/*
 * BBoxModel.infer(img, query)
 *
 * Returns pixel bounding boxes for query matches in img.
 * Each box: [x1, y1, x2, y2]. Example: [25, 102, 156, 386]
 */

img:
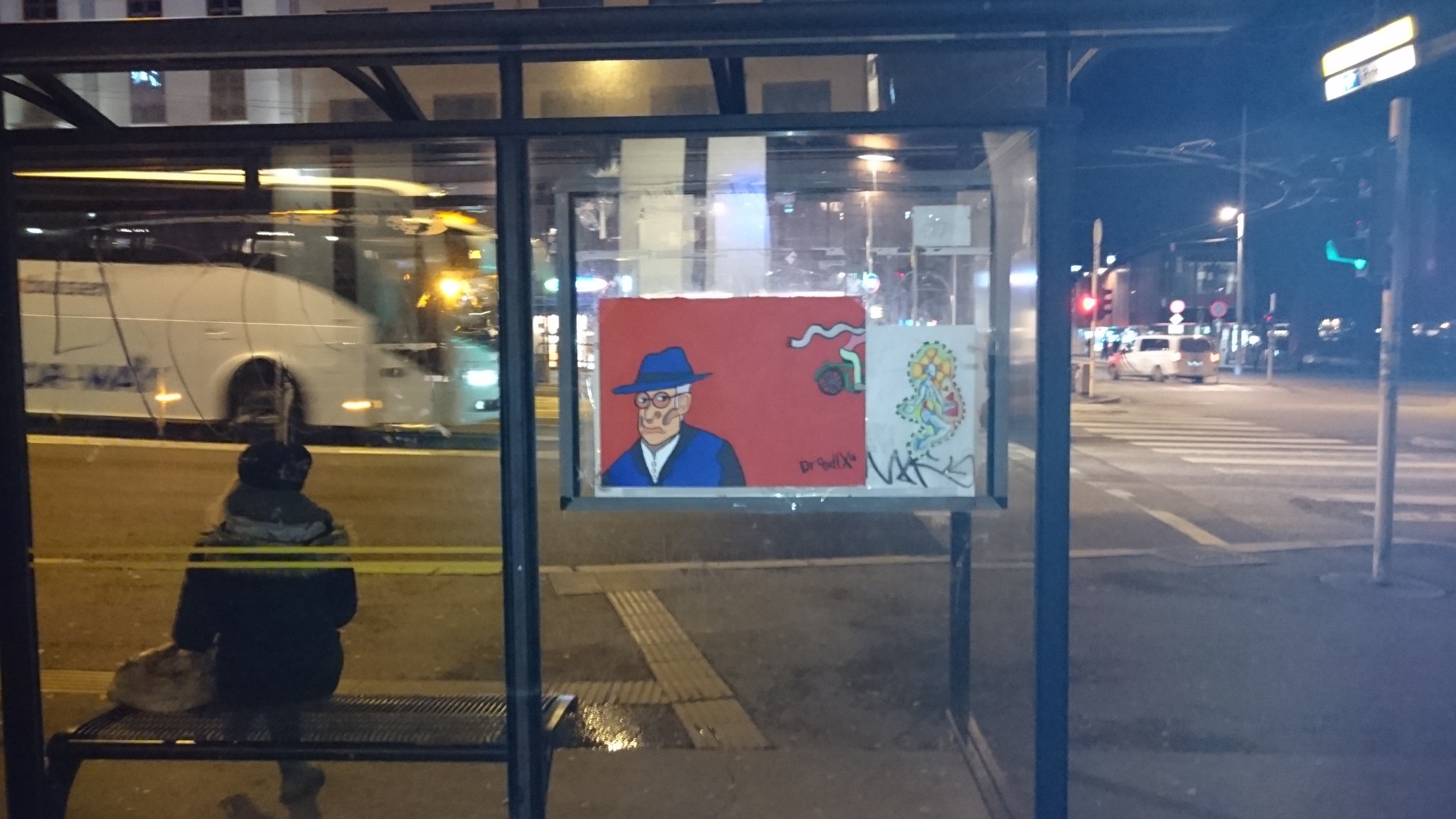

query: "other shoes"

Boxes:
[280, 765, 324, 800]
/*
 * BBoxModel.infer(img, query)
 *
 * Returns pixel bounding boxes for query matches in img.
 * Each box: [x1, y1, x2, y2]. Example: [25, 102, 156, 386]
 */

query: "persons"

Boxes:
[176, 448, 357, 809]
[1099, 340, 1109, 361]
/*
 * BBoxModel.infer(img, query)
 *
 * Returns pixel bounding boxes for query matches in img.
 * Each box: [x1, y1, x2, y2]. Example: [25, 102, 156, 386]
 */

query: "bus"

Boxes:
[1, 165, 562, 449]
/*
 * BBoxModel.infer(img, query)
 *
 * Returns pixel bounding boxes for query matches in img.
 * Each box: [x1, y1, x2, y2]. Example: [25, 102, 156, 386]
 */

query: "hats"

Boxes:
[237, 440, 312, 490]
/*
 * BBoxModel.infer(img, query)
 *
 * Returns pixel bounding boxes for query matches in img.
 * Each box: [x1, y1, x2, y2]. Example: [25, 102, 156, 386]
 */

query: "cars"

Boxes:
[1070, 326, 1161, 386]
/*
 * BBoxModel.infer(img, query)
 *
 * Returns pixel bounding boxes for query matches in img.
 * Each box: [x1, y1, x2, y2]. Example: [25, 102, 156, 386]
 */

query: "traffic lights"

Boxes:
[1096, 286, 1113, 326]
[1316, 138, 1394, 288]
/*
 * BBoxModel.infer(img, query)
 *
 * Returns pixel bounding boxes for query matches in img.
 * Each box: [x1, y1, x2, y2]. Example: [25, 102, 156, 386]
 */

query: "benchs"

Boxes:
[47, 692, 580, 819]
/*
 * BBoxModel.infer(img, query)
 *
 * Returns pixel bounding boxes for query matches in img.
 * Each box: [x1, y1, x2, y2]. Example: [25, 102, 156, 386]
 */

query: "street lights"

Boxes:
[1317, 15, 1421, 584]
[1216, 206, 1247, 374]
[1069, 216, 1115, 398]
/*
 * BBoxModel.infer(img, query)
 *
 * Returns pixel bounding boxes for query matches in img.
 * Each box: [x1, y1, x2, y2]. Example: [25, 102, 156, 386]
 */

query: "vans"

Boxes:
[1105, 333, 1219, 384]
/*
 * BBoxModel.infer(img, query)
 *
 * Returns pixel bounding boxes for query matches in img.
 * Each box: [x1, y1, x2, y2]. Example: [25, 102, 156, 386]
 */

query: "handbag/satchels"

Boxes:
[107, 641, 216, 712]
